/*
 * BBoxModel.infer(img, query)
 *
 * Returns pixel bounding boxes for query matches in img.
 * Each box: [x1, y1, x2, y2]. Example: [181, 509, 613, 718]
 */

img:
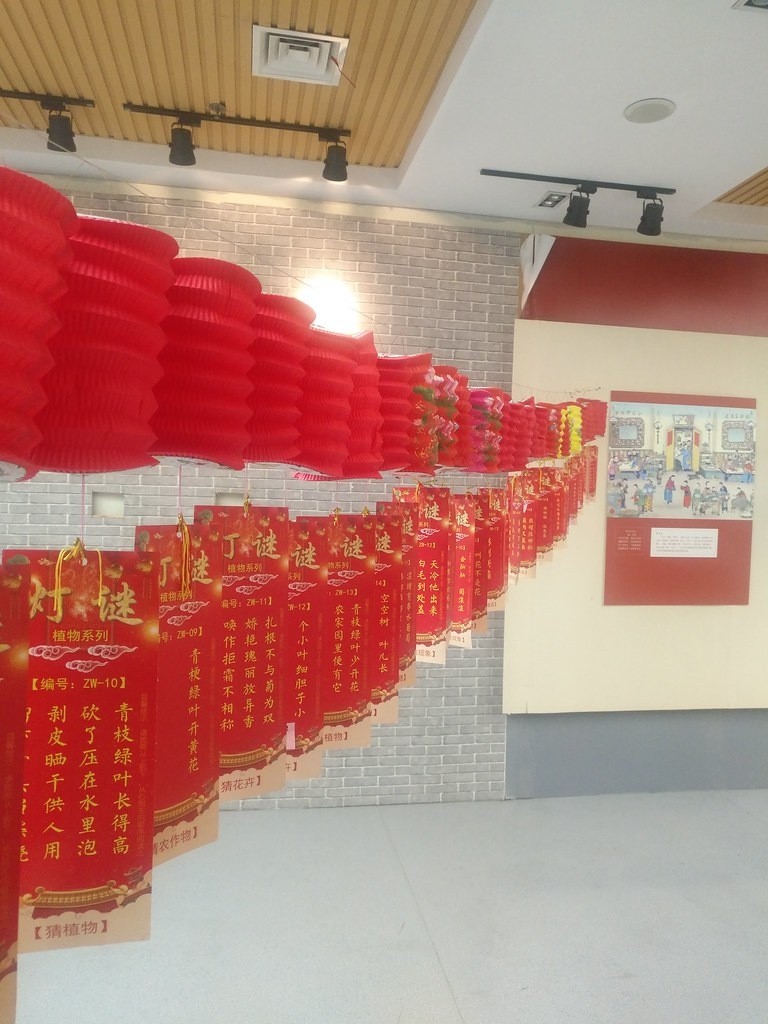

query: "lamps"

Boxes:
[168, 115, 200, 166]
[40, 100, 76, 152]
[317, 132, 348, 181]
[562, 183, 596, 229]
[634, 188, 664, 236]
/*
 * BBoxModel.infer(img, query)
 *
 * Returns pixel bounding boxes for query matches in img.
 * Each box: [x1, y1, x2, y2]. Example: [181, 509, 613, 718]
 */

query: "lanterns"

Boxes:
[0, 165, 608, 481]
